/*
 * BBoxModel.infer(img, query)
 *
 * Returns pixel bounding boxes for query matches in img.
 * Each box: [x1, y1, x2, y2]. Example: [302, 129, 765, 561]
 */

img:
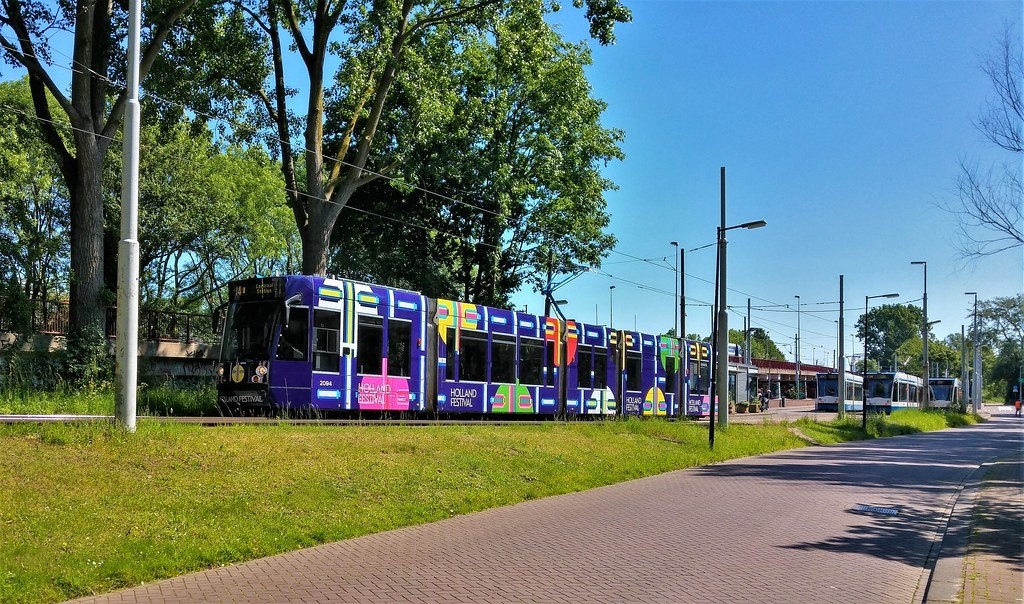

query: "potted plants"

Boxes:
[736, 400, 749, 413]
[748, 399, 762, 413]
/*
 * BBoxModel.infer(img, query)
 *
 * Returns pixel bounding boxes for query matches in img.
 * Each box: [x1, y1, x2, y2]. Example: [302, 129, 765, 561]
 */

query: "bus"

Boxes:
[862, 369, 923, 414]
[211, 266, 719, 419]
[814, 367, 863, 412]
[929, 377, 963, 411]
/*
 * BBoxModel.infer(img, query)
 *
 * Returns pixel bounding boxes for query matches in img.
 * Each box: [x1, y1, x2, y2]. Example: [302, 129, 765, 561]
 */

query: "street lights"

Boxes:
[610, 286, 616, 328]
[821, 345, 825, 365]
[964, 292, 978, 414]
[709, 219, 767, 449]
[670, 241, 679, 337]
[813, 348, 816, 365]
[795, 295, 801, 400]
[910, 261, 930, 409]
[862, 293, 900, 429]
[835, 321, 842, 375]
[851, 333, 854, 374]
[1019, 366, 1024, 403]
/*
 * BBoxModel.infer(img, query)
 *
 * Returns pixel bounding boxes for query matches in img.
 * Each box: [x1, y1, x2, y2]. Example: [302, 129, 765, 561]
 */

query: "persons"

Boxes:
[1014, 399, 1023, 416]
[758, 388, 772, 412]
